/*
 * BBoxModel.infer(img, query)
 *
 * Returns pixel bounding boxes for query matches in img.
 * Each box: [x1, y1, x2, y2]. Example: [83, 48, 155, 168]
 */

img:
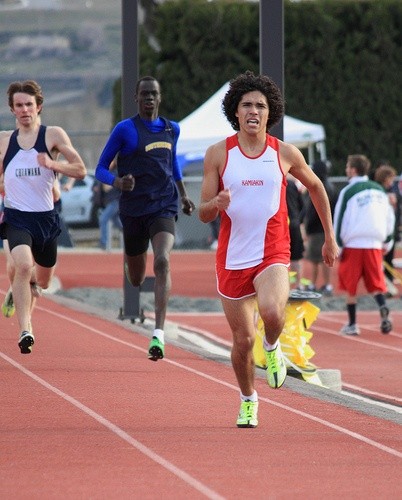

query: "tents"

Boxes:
[170, 79, 328, 249]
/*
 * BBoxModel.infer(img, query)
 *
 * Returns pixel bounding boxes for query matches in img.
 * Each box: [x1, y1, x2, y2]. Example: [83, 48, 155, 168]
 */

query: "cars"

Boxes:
[58, 169, 104, 228]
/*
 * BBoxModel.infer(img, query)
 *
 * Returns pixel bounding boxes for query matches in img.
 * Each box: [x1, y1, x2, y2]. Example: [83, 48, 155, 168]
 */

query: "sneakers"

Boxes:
[2, 287, 17, 317]
[236, 400, 259, 428]
[379, 304, 392, 334]
[338, 325, 360, 337]
[264, 334, 287, 389]
[146, 336, 165, 362]
[18, 331, 35, 354]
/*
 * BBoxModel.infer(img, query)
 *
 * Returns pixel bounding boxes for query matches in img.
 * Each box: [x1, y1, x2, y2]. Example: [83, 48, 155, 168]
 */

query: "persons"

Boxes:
[0, 81, 86, 354]
[372, 164, 397, 297]
[0, 108, 75, 318]
[267, 161, 309, 295]
[301, 160, 336, 292]
[92, 157, 124, 250]
[94, 74, 196, 362]
[196, 72, 340, 427]
[331, 154, 394, 335]
